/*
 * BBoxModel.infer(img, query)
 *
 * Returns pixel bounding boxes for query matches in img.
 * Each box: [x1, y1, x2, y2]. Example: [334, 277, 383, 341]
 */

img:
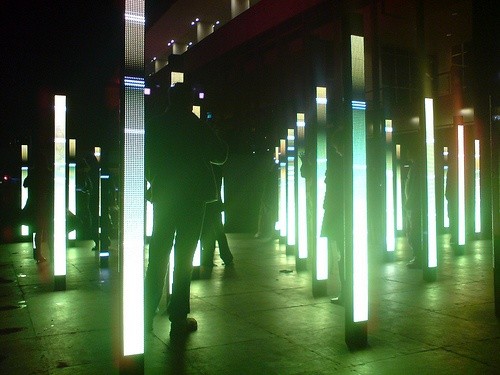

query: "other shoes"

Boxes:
[330, 297, 341, 305]
[170, 317, 198, 338]
[407, 256, 418, 268]
[92, 245, 99, 251]
[224, 259, 233, 265]
[203, 265, 213, 278]
[253, 232, 267, 239]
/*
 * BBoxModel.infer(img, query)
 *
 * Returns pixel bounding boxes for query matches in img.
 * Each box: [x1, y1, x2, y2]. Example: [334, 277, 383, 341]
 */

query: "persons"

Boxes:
[14, 147, 115, 263]
[202, 104, 466, 304]
[143, 82, 230, 338]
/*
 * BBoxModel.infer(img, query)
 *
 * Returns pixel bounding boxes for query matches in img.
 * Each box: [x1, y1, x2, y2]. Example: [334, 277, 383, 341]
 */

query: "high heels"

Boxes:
[34, 256, 47, 264]
